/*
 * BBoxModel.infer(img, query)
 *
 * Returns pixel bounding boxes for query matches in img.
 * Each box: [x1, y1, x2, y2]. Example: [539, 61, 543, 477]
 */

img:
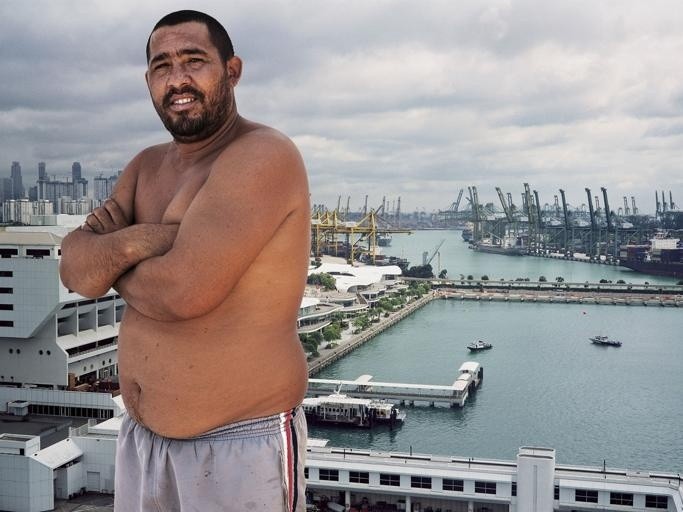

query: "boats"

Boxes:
[467, 340, 492, 352]
[302, 393, 407, 428]
[619, 231, 683, 277]
[366, 232, 392, 246]
[473, 230, 528, 256]
[588, 335, 622, 347]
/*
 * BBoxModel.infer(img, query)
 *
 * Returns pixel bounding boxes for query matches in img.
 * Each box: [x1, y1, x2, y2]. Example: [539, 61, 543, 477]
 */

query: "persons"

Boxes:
[55, 8, 311, 512]
